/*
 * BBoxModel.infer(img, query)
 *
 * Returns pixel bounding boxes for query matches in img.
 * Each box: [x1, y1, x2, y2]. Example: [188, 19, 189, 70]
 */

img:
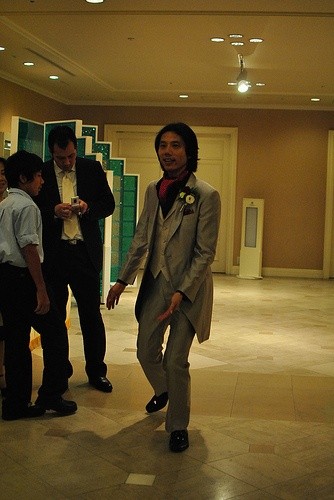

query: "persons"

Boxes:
[0, 150, 78, 421]
[106, 122, 222, 453]
[31, 126, 116, 396]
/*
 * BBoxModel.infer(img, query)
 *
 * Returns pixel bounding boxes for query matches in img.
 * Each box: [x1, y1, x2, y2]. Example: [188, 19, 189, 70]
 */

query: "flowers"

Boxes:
[178, 191, 195, 206]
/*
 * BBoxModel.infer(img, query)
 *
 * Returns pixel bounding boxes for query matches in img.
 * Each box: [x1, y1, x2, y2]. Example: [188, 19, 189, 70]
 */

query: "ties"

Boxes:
[61, 170, 80, 240]
[157, 175, 178, 201]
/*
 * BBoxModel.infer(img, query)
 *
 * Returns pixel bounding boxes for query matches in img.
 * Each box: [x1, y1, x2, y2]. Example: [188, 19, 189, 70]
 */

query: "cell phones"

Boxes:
[70, 196, 79, 206]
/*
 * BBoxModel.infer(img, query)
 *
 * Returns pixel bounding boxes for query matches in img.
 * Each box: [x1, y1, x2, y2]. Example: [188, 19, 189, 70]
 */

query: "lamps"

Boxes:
[236, 54, 249, 93]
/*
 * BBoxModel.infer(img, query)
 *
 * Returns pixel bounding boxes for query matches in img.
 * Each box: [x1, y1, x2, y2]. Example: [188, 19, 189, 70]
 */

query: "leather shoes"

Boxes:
[88, 375, 113, 393]
[35, 394, 77, 415]
[145, 392, 168, 413]
[169, 430, 189, 452]
[60, 378, 68, 394]
[2, 400, 42, 420]
